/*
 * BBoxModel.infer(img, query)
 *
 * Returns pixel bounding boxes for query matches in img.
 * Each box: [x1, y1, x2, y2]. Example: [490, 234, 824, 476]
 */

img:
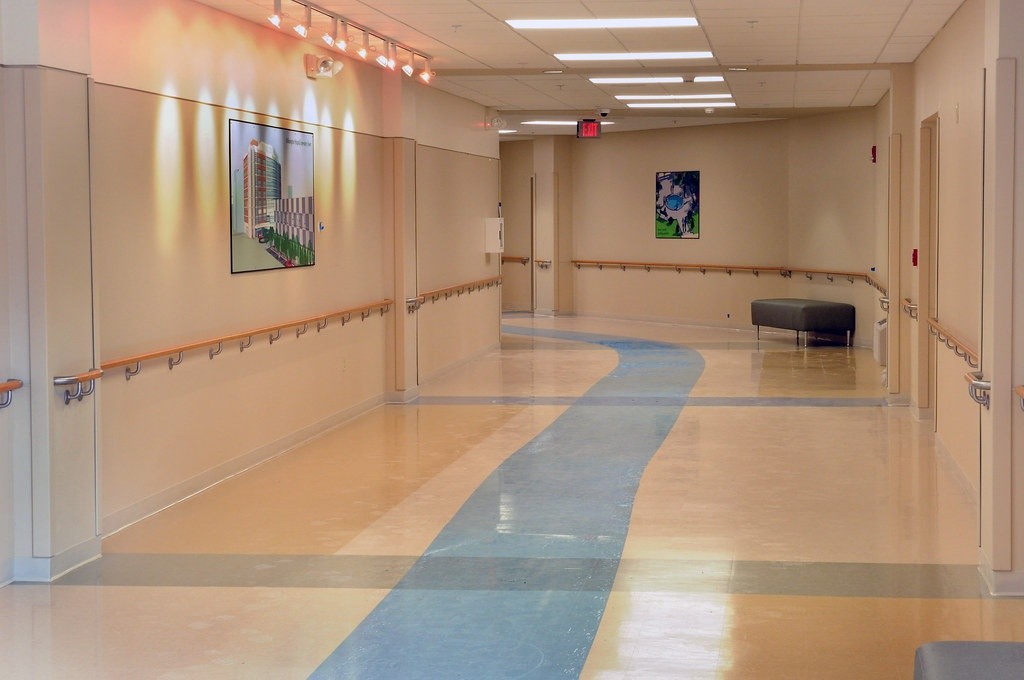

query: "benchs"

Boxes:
[751, 298, 856, 347]
[912, 641, 1024, 680]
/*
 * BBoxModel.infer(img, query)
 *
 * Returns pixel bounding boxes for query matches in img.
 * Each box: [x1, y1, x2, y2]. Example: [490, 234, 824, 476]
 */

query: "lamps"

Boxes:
[376, 40, 389, 66]
[388, 44, 398, 70]
[402, 52, 415, 77]
[268, 0, 285, 28]
[304, 54, 344, 79]
[322, 18, 338, 48]
[293, 6, 312, 38]
[356, 31, 370, 59]
[336, 21, 349, 52]
[419, 59, 436, 84]
